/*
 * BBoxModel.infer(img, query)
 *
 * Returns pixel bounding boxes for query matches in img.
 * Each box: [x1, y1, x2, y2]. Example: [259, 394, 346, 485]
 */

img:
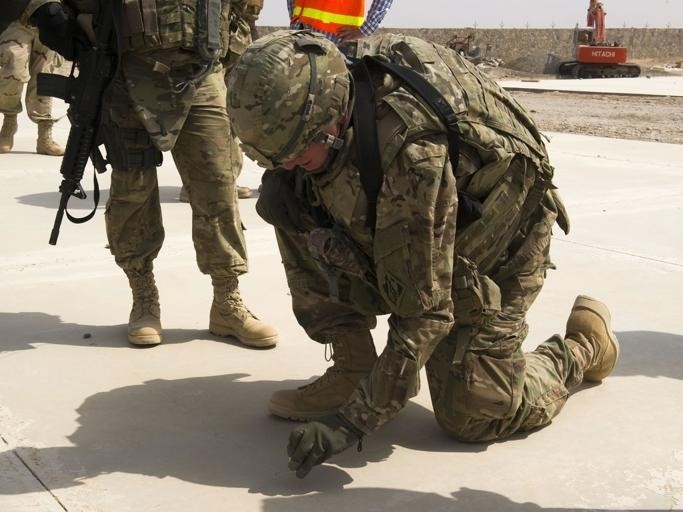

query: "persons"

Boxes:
[1, 1, 66, 154]
[180, 0, 264, 203]
[9, 0, 279, 348]
[225, 26, 620, 477]
[259, 0, 392, 192]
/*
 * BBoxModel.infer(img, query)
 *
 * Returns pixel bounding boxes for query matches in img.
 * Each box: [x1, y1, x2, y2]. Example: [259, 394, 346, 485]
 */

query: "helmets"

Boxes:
[225, 28, 351, 170]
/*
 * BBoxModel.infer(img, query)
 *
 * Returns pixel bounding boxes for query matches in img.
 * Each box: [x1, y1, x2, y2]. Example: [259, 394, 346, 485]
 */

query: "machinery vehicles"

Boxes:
[554, 1, 641, 78]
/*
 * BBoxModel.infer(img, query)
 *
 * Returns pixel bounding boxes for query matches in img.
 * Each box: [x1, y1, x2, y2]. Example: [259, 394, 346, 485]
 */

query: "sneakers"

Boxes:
[180, 179, 263, 203]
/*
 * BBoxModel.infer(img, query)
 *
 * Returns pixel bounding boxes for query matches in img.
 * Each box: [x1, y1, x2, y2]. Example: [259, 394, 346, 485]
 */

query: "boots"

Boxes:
[0, 113, 18, 153]
[36, 121, 65, 155]
[126, 271, 164, 347]
[208, 276, 280, 348]
[563, 294, 620, 383]
[268, 327, 379, 423]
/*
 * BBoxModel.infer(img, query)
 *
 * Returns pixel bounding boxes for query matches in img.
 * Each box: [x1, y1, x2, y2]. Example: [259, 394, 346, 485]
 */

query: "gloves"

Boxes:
[37, 2, 76, 62]
[286, 412, 364, 479]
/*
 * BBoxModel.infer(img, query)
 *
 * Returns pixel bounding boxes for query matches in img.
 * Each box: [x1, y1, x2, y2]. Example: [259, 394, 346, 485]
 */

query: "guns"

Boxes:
[36, 0, 122, 245]
